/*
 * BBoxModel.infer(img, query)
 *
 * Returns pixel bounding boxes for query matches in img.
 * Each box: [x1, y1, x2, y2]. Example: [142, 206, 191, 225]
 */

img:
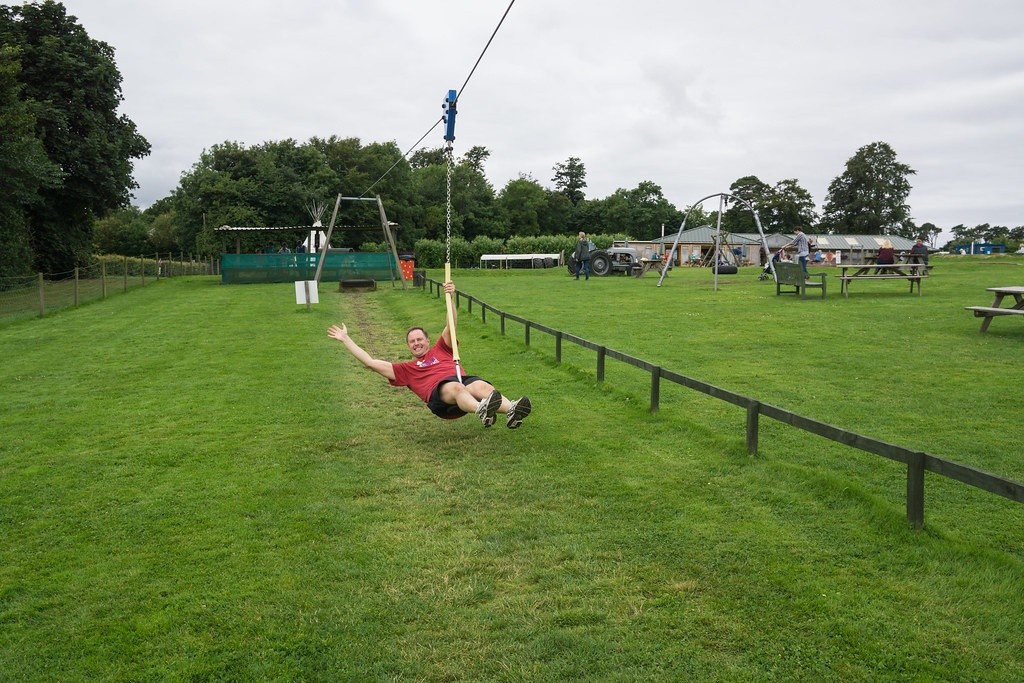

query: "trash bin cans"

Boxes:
[399, 254, 415, 279]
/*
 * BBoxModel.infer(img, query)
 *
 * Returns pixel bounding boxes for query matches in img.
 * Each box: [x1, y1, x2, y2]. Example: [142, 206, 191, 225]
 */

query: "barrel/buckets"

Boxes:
[399, 255, 415, 280]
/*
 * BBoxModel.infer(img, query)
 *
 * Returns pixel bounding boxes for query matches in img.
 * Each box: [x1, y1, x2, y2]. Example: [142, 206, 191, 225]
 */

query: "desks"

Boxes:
[854, 256, 907, 276]
[637, 260, 668, 279]
[837, 264, 934, 296]
[893, 254, 925, 268]
[980, 287, 1024, 332]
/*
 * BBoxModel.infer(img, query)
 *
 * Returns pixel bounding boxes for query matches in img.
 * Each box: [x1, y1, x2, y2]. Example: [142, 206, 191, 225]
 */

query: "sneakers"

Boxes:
[477, 390, 502, 428]
[506, 396, 532, 429]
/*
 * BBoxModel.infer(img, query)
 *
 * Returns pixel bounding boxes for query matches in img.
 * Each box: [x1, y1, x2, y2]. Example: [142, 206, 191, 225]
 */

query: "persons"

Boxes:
[909, 238, 929, 276]
[327, 281, 532, 430]
[254, 239, 308, 278]
[649, 251, 697, 267]
[1020, 243, 1024, 254]
[873, 240, 894, 275]
[574, 232, 591, 281]
[778, 225, 834, 279]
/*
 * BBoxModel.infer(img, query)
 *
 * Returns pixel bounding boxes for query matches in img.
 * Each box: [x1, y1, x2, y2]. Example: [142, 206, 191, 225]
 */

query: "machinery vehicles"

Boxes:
[568, 237, 643, 279]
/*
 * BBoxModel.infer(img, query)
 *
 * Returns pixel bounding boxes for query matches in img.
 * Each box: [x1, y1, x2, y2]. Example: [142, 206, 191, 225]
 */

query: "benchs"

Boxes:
[632, 267, 642, 269]
[663, 266, 672, 269]
[774, 259, 827, 300]
[964, 306, 1024, 315]
[834, 274, 929, 279]
